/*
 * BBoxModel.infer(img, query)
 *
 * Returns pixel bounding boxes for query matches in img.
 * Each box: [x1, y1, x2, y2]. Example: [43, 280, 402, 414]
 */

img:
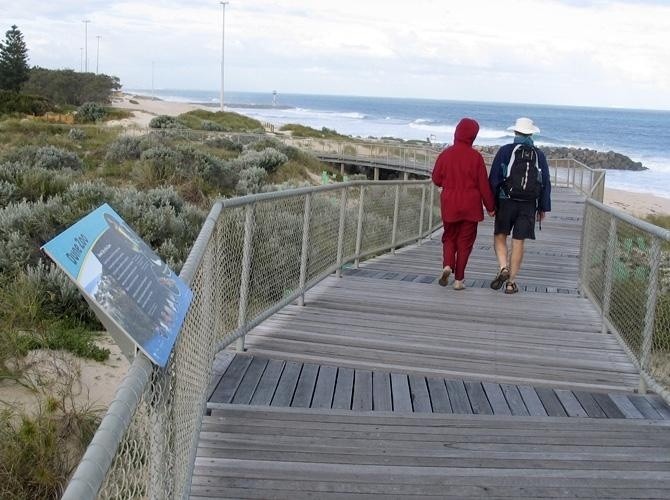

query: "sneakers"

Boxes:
[490, 266, 511, 289]
[507, 117, 541, 136]
[504, 281, 519, 293]
[453, 280, 467, 290]
[438, 266, 452, 287]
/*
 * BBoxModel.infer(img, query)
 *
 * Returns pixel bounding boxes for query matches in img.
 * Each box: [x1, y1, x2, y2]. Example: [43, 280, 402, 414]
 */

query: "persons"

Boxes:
[432, 118, 495, 290]
[489, 118, 551, 294]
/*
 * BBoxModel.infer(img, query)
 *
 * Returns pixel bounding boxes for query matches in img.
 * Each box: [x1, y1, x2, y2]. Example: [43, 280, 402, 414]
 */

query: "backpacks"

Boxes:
[498, 146, 544, 201]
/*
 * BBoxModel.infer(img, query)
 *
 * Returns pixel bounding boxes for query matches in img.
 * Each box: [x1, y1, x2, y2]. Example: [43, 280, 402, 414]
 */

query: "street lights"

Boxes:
[78, 46, 84, 71]
[81, 19, 91, 73]
[95, 35, 102, 74]
[216, 0, 230, 111]
